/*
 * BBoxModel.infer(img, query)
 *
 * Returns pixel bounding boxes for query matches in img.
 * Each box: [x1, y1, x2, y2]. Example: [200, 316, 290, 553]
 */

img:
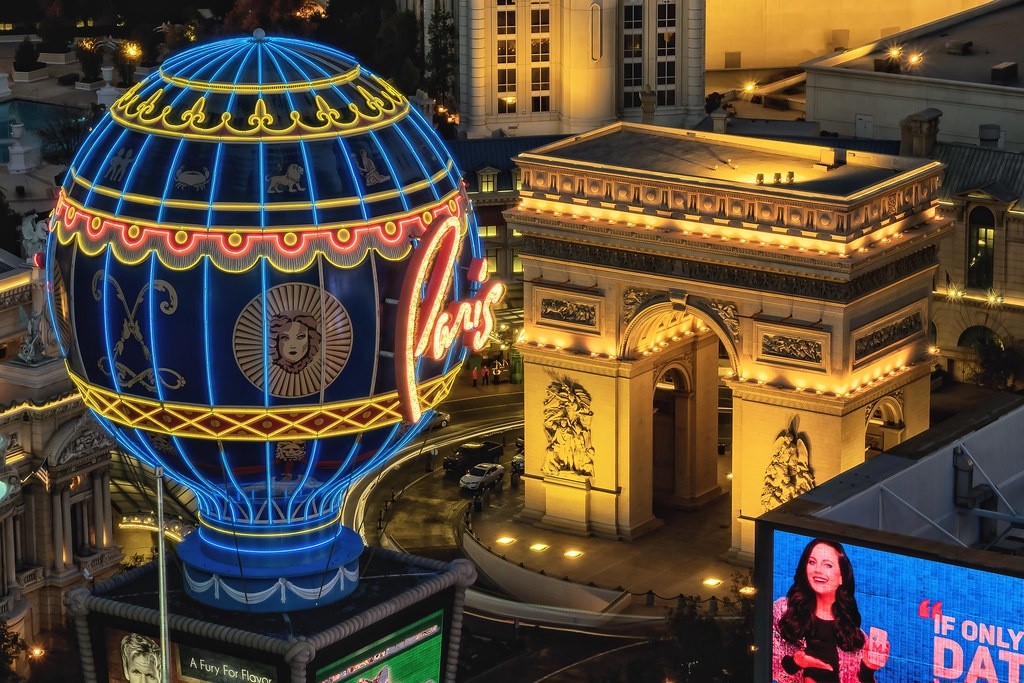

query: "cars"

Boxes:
[420, 410, 451, 433]
[460, 463, 504, 494]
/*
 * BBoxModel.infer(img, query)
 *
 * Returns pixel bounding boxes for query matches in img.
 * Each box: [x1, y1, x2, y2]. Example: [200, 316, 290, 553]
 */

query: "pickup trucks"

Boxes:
[443, 440, 504, 475]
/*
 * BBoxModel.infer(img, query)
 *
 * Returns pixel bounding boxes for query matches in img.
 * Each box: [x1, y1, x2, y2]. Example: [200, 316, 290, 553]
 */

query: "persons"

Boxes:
[772, 538, 890, 683]
[481, 365, 490, 386]
[120, 633, 162, 683]
[472, 366, 479, 387]
